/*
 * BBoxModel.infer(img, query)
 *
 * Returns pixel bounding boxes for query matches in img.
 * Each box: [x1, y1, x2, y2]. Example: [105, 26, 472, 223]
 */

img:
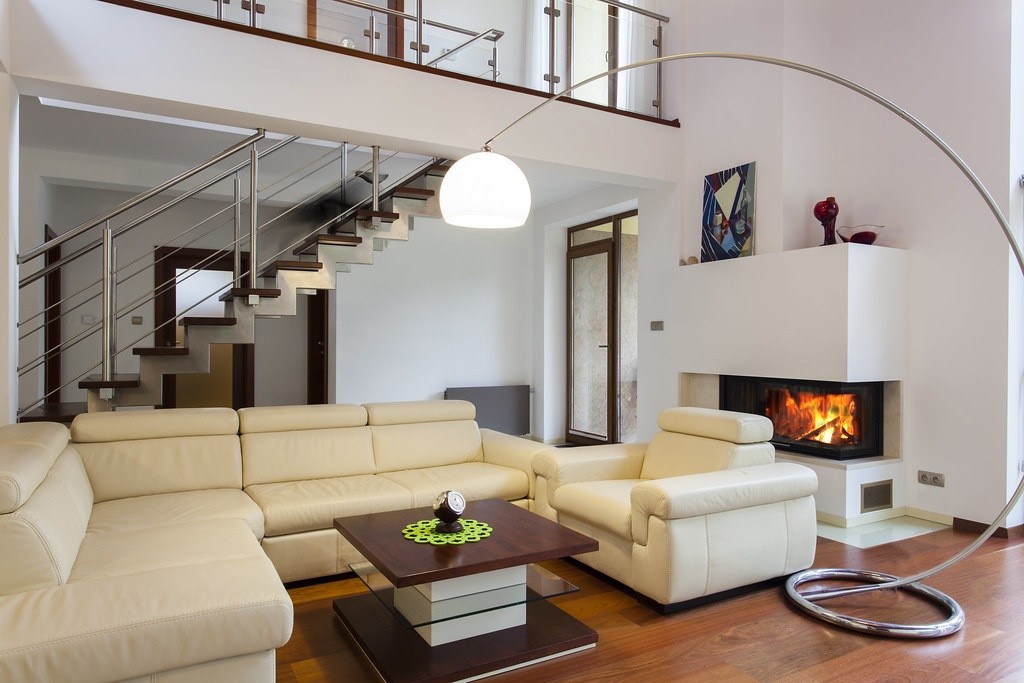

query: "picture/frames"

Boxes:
[700, 160, 757, 263]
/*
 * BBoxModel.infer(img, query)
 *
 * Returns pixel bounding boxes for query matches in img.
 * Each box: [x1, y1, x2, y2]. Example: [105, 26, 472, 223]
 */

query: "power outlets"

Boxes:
[917, 470, 945, 488]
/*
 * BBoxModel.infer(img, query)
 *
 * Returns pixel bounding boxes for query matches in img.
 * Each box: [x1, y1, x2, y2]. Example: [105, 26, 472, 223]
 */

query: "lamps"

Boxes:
[439, 52, 1024, 638]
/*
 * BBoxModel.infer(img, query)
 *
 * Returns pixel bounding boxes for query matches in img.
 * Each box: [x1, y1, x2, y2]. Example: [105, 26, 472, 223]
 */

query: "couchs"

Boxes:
[0, 394, 817, 683]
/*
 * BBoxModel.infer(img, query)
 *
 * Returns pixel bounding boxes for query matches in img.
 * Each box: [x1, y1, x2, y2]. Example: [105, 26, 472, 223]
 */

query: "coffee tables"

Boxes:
[332, 497, 599, 683]
